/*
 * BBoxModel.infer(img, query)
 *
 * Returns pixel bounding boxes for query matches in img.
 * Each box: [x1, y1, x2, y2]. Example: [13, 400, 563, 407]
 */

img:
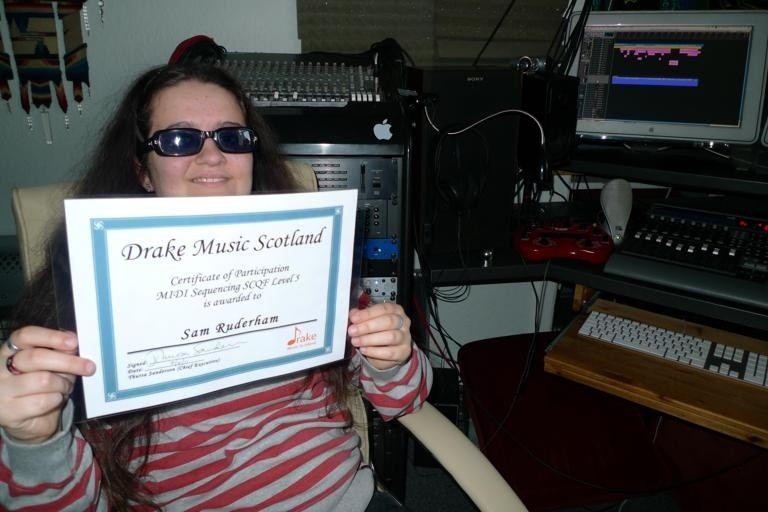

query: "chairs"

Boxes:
[6, 159, 537, 512]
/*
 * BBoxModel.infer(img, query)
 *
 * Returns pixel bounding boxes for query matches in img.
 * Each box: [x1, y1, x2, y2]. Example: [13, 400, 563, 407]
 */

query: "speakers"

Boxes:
[409, 67, 523, 269]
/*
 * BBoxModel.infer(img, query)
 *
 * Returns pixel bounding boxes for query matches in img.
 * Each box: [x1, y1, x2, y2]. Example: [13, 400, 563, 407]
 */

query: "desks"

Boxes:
[507, 199, 765, 451]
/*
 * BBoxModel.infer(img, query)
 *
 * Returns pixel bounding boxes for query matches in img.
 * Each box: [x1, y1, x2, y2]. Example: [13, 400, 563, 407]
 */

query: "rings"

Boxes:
[396, 313, 407, 332]
[5, 332, 22, 352]
[4, 352, 26, 373]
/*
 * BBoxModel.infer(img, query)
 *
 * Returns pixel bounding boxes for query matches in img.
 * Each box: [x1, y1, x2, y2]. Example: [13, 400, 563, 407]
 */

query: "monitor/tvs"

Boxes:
[569, 9, 768, 144]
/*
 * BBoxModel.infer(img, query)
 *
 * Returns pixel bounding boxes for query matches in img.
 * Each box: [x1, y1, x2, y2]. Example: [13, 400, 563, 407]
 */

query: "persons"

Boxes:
[2, 62, 442, 511]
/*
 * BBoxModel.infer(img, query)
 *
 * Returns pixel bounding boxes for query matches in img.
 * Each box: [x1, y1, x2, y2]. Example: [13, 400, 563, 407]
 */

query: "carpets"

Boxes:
[456, 331, 765, 510]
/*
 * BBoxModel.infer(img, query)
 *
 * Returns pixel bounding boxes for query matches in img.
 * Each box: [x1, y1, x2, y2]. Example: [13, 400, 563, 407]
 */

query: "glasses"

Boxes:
[144, 125, 261, 157]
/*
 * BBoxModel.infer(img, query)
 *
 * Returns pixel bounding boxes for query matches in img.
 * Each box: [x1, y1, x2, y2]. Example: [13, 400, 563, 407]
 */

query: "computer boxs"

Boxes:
[415, 368, 460, 466]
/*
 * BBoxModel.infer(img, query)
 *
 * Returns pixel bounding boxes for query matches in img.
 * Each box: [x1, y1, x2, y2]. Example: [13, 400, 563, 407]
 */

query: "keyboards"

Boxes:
[578, 311, 768, 389]
